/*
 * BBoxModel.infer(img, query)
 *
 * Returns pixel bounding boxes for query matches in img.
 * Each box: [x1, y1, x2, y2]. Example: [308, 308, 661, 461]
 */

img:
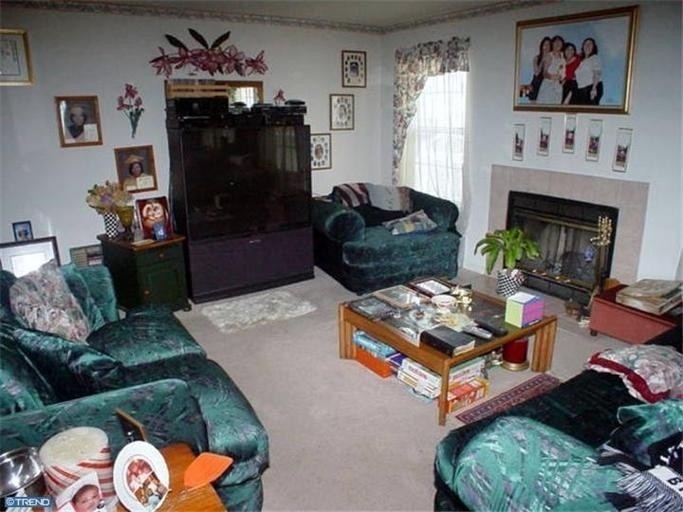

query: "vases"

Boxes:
[101, 209, 119, 239]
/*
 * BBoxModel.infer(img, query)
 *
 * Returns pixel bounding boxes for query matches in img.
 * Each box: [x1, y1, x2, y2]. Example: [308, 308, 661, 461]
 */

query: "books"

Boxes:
[418, 325, 476, 357]
[614, 278, 682, 317]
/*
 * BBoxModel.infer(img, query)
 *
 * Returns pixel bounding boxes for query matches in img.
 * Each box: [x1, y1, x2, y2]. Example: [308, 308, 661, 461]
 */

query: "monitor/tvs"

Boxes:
[165, 114, 312, 242]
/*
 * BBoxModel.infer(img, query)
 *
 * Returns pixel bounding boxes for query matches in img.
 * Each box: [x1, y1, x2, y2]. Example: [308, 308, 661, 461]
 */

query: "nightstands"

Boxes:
[97, 232, 191, 318]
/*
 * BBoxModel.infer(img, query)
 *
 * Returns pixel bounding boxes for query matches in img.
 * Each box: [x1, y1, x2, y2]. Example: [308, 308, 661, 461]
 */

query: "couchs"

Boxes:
[0, 263, 270, 511]
[433, 322, 683, 511]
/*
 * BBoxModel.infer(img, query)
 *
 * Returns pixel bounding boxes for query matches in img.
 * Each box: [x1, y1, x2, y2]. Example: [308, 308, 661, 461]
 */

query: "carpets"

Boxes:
[454, 372, 564, 426]
[202, 288, 319, 334]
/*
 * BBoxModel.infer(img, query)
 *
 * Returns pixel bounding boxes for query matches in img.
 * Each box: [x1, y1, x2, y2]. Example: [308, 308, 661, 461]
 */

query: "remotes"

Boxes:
[463, 325, 492, 339]
[477, 322, 508, 336]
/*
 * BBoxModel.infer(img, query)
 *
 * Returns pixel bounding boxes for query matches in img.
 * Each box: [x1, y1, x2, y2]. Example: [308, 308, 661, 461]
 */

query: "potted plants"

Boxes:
[473, 225, 542, 297]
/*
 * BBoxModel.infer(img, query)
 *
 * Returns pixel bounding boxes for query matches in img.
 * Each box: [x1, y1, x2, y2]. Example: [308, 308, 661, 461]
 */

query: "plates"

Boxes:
[431, 294, 456, 305]
[110, 439, 171, 512]
[408, 309, 432, 323]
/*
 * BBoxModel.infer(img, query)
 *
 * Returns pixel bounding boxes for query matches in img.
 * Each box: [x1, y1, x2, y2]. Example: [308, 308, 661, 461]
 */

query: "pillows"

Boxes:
[584, 343, 683, 403]
[13, 258, 126, 393]
[606, 400, 683, 466]
[382, 209, 438, 236]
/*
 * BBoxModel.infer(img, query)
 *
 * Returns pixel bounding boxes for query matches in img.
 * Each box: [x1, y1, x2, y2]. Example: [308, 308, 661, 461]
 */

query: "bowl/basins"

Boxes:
[0, 446, 47, 512]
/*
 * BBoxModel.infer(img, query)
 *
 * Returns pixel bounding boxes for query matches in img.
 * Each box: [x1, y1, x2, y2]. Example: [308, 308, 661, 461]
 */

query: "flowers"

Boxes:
[148, 27, 269, 78]
[116, 83, 145, 138]
[84, 179, 132, 215]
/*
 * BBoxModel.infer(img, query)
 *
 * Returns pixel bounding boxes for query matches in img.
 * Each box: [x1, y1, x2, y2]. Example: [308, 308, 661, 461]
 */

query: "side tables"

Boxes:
[30, 442, 227, 512]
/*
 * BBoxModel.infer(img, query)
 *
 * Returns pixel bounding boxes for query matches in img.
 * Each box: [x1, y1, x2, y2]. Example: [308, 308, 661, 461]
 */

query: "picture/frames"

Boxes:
[1, 28, 36, 87]
[512, 4, 639, 115]
[114, 144, 158, 193]
[347, 296, 397, 322]
[407, 274, 453, 298]
[373, 283, 430, 312]
[328, 93, 355, 131]
[12, 220, 33, 241]
[311, 132, 333, 170]
[115, 407, 147, 440]
[511, 114, 633, 173]
[1, 235, 61, 280]
[341, 49, 367, 88]
[53, 93, 103, 148]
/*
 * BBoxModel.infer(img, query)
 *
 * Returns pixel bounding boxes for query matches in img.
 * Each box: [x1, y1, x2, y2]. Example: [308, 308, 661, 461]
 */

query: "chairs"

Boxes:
[312, 181, 462, 296]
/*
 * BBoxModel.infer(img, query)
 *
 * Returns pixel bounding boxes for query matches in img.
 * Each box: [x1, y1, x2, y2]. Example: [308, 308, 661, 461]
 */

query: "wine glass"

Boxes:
[114, 205, 135, 241]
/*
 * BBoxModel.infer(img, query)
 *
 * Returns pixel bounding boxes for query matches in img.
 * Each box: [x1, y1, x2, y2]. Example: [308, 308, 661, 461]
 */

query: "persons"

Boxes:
[559, 42, 583, 106]
[573, 38, 603, 106]
[64, 105, 87, 138]
[70, 485, 100, 510]
[120, 154, 146, 189]
[535, 33, 567, 105]
[352, 329, 485, 399]
[125, 462, 166, 510]
[518, 34, 564, 104]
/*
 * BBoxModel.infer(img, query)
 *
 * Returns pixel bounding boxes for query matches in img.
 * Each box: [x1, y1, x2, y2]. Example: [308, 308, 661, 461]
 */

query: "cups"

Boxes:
[457, 288, 473, 316]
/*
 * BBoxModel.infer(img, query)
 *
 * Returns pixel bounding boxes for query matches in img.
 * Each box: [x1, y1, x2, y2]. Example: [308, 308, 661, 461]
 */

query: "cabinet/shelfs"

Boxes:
[165, 114, 315, 304]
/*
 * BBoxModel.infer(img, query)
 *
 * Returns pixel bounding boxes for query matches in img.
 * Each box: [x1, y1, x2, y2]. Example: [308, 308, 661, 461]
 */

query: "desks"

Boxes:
[588, 284, 683, 344]
